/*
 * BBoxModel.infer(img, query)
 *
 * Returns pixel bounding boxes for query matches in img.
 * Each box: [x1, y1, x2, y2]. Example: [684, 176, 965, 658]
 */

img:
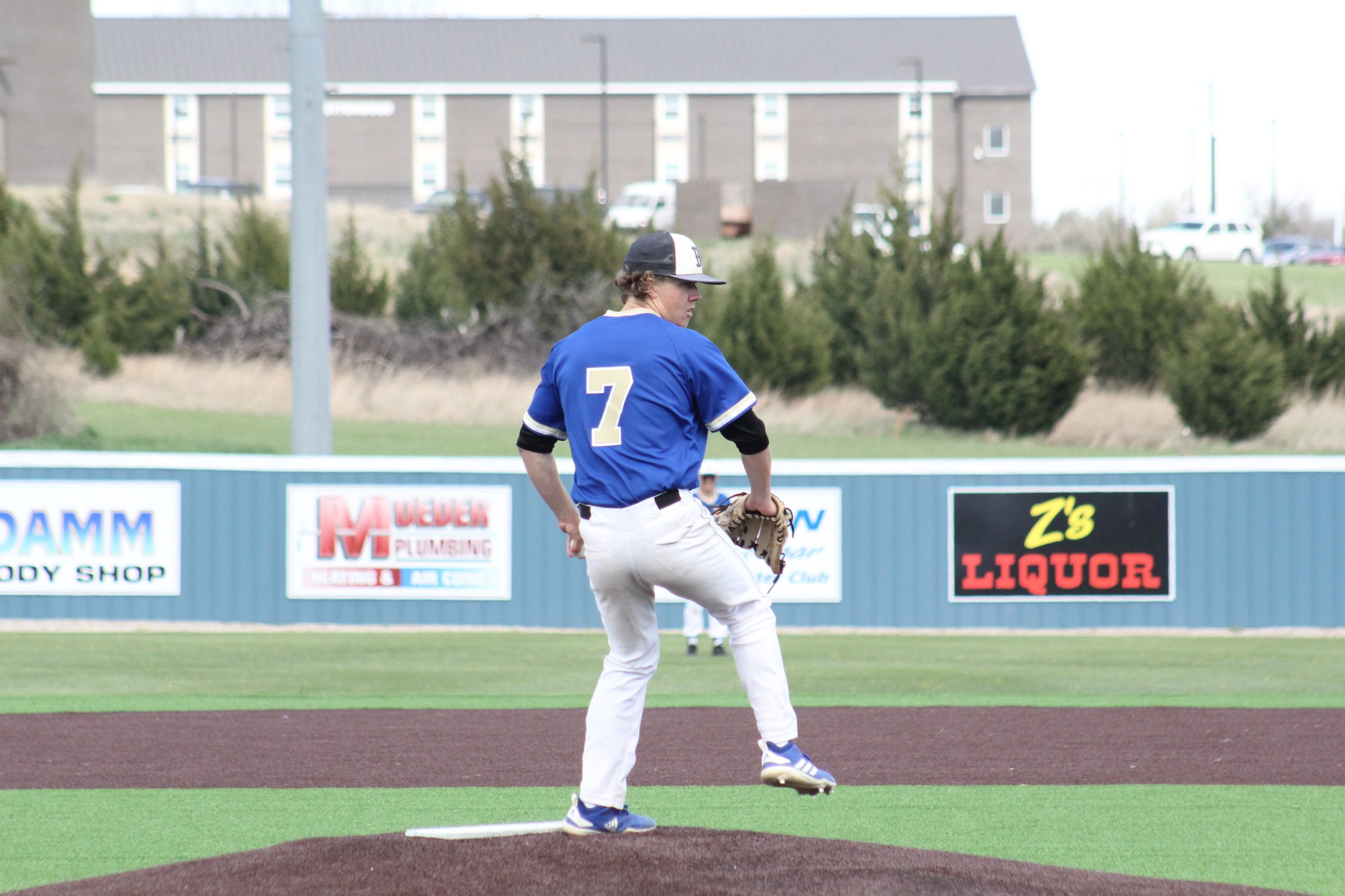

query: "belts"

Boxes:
[578, 488, 681, 520]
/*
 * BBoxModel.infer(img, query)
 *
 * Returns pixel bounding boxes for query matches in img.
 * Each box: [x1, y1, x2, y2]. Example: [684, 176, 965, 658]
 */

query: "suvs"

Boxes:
[1137, 204, 1264, 265]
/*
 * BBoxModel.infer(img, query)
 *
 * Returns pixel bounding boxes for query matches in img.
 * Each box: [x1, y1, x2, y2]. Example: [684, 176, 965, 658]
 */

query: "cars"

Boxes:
[1261, 234, 1345, 268]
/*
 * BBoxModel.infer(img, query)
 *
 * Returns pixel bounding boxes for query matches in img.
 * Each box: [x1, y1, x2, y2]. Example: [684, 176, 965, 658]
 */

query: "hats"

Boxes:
[623, 232, 727, 285]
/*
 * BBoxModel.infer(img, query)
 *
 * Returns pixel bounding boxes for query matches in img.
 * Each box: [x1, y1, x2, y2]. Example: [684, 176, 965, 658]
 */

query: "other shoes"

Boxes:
[688, 642, 696, 654]
[713, 644, 724, 655]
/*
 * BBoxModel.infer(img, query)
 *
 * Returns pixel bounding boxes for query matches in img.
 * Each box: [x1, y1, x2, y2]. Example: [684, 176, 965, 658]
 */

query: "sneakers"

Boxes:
[758, 740, 836, 798]
[561, 793, 656, 836]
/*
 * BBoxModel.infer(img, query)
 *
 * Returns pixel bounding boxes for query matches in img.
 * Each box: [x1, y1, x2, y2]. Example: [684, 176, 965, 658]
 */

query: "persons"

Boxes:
[515, 231, 836, 836]
[676, 465, 730, 654]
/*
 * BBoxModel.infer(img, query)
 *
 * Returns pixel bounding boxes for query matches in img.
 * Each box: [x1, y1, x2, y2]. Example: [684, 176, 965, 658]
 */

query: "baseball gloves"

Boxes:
[704, 492, 796, 595]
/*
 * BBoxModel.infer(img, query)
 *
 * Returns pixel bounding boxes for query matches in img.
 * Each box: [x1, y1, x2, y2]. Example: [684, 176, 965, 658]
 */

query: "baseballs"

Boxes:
[570, 539, 586, 560]
